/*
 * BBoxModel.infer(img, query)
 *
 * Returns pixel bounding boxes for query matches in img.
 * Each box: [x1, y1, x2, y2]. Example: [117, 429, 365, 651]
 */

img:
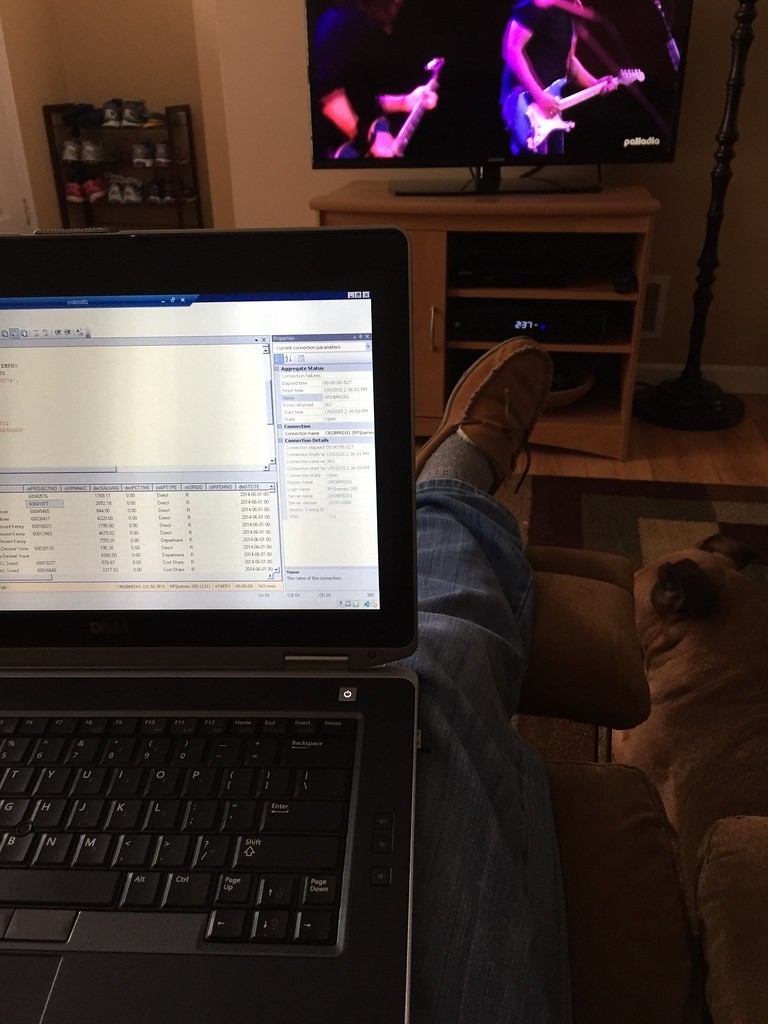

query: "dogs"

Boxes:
[609, 532, 768, 873]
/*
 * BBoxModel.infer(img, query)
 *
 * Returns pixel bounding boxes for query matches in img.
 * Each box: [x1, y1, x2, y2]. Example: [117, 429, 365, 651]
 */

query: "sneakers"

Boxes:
[65, 177, 88, 203]
[132, 138, 155, 167]
[103, 98, 121, 127]
[156, 138, 190, 164]
[163, 176, 179, 203]
[146, 176, 162, 205]
[82, 140, 119, 162]
[109, 174, 124, 205]
[125, 176, 143, 205]
[121, 98, 147, 126]
[83, 178, 108, 204]
[182, 181, 197, 203]
[61, 140, 81, 161]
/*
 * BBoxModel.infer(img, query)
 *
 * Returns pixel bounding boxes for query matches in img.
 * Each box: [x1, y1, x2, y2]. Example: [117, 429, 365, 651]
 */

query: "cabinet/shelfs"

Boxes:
[308, 175, 664, 460]
[42, 102, 207, 230]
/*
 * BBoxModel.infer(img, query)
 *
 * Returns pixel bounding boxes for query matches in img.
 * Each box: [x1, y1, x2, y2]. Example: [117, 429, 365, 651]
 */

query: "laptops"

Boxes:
[0, 224, 419, 1024]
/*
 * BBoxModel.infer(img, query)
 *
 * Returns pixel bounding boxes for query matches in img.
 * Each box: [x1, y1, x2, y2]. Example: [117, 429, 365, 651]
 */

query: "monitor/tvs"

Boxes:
[306, 0, 694, 196]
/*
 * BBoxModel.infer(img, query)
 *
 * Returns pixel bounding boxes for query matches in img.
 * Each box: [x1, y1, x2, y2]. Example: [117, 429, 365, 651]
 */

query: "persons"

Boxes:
[500, 0, 614, 154]
[390, 334, 564, 1023]
[315, 0, 438, 160]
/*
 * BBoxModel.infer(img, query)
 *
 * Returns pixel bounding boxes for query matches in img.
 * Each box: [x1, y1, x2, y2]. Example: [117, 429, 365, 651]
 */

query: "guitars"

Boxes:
[329, 56, 446, 159]
[508, 68, 645, 152]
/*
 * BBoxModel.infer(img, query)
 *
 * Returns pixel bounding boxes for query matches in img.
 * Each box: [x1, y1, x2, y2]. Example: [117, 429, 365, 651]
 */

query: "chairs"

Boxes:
[508, 539, 768, 1024]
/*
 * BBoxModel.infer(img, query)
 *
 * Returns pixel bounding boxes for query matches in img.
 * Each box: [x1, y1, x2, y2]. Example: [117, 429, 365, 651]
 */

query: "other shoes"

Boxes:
[415, 336, 554, 497]
[61, 104, 103, 125]
[142, 112, 167, 128]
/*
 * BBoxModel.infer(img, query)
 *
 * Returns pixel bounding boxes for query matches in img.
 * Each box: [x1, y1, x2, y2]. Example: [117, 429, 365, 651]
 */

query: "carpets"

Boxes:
[486, 470, 768, 765]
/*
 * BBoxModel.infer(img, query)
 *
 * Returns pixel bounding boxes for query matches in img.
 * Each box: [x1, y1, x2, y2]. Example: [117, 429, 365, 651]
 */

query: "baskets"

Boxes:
[542, 367, 592, 413]
